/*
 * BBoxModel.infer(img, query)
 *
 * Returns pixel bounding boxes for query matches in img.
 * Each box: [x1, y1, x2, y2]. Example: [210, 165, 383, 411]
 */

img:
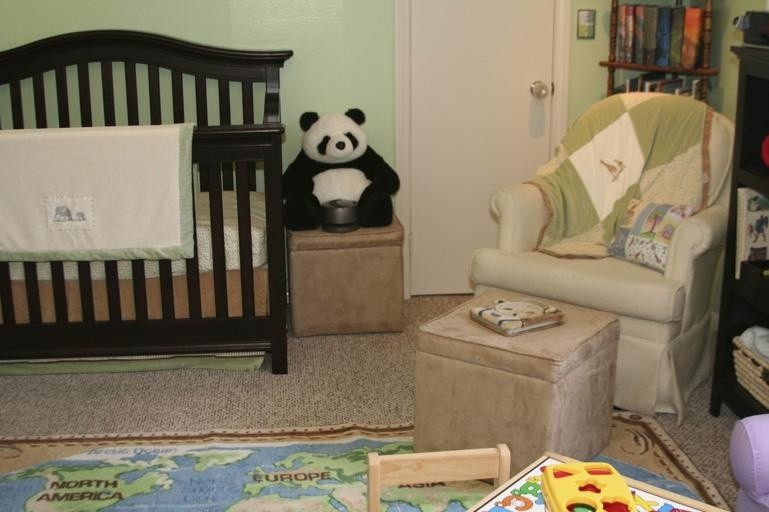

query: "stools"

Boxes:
[286, 212, 405, 338]
[411, 290, 621, 485]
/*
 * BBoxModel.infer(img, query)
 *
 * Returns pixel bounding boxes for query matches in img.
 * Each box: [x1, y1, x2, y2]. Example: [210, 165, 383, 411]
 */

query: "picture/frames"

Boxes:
[577, 9, 596, 39]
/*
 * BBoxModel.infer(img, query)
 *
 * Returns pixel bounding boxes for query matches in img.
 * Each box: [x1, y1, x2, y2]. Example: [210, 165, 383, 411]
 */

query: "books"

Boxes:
[614, 4, 712, 102]
[469, 297, 566, 337]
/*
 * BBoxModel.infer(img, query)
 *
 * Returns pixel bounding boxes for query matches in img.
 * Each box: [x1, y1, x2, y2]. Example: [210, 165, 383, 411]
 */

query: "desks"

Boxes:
[465, 450, 730, 512]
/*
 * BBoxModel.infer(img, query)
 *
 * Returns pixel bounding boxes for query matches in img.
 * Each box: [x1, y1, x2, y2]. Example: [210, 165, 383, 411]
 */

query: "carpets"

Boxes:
[18, 287, 479, 445]
[0, 408, 732, 512]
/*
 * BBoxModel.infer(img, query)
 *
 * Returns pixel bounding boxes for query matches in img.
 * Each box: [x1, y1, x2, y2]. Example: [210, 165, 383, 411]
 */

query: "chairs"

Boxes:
[728, 413, 768, 511]
[468, 90, 735, 427]
[367, 442, 511, 511]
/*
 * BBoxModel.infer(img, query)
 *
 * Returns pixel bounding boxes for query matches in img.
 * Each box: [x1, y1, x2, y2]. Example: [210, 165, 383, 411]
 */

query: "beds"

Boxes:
[1, 29, 289, 375]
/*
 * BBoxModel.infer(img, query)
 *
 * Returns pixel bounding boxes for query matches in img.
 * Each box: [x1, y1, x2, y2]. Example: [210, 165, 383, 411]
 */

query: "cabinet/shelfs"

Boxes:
[710, 45, 768, 418]
[598, 0, 717, 106]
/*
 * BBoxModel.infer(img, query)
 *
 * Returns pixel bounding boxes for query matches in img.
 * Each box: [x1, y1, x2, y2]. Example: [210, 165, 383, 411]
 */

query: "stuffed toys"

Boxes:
[283, 106, 400, 232]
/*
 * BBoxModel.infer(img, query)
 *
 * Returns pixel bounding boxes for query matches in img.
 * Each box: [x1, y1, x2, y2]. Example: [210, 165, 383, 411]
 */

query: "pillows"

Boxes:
[606, 197, 699, 274]
